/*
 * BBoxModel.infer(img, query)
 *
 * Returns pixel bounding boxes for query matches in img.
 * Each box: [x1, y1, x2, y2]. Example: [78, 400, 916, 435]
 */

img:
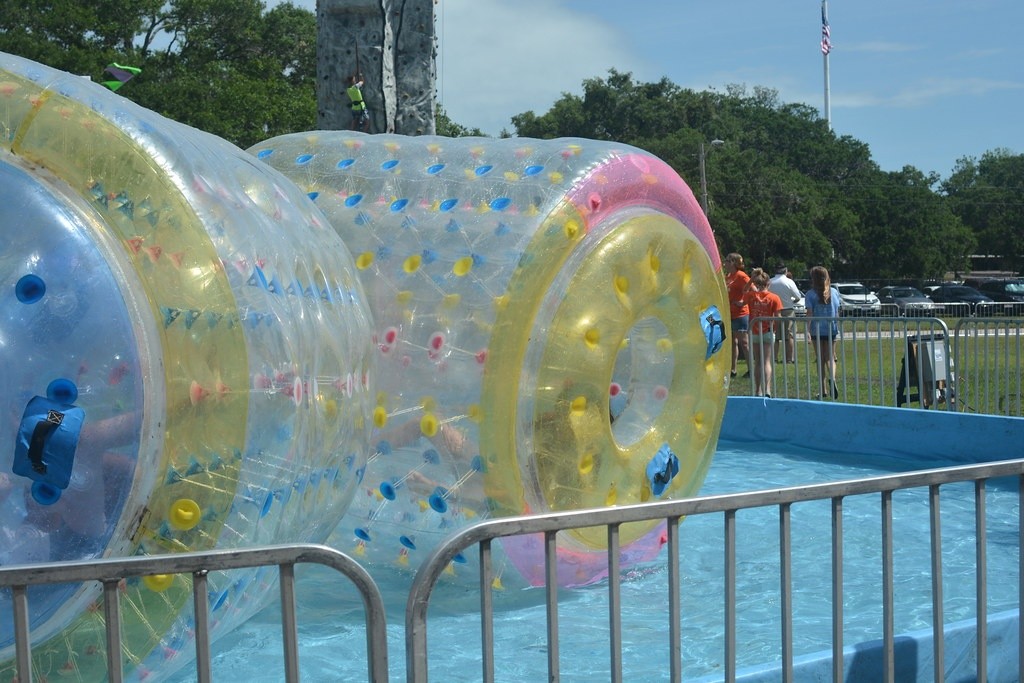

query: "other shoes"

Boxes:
[743, 370, 750, 378]
[731, 371, 737, 378]
[816, 393, 828, 399]
[829, 379, 838, 399]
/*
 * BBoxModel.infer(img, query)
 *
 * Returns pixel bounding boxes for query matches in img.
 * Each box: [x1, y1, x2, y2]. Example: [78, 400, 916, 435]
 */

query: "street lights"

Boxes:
[698, 140, 725, 215]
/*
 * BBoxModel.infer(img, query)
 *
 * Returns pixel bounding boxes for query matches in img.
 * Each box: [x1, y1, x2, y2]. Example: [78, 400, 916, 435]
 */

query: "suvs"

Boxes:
[830, 282, 881, 317]
[963, 276, 1024, 316]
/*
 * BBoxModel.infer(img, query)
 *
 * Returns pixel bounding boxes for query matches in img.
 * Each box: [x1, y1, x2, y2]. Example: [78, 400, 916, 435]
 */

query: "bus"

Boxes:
[943, 254, 1020, 281]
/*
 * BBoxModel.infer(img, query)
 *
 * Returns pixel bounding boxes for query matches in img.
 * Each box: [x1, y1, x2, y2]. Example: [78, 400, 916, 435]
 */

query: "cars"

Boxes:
[791, 290, 807, 316]
[930, 286, 995, 318]
[876, 286, 934, 316]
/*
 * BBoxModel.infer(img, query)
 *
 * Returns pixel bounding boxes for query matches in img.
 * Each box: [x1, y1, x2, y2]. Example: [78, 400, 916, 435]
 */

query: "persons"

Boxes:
[831, 287, 842, 363]
[364, 378, 615, 503]
[0, 396, 185, 591]
[733, 266, 784, 397]
[345, 69, 369, 133]
[804, 265, 840, 399]
[724, 252, 758, 378]
[767, 262, 801, 363]
